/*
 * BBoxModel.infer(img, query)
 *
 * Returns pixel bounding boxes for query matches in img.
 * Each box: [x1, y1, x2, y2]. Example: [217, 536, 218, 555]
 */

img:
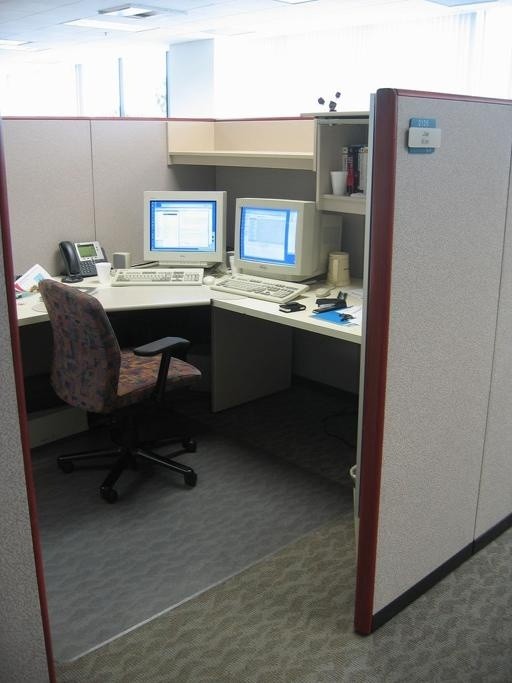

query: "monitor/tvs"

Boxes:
[234, 197, 343, 283]
[143, 190, 227, 268]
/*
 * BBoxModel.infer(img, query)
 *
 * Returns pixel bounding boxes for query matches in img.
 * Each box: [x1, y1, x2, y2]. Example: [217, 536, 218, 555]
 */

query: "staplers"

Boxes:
[312, 298, 346, 313]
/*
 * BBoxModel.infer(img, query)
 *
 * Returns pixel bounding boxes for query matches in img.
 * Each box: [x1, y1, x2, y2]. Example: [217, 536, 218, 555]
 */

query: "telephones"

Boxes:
[59, 241, 108, 277]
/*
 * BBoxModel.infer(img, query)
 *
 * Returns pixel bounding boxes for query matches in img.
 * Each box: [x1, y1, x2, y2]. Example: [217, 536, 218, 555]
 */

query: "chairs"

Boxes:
[37, 276, 206, 503]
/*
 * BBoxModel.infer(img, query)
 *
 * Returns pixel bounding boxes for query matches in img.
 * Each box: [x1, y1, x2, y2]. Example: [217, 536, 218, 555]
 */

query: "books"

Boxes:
[342, 145, 368, 198]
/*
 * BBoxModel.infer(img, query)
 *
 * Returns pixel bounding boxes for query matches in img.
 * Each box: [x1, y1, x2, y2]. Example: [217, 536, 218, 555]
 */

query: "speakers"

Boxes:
[226, 251, 235, 267]
[112, 252, 132, 269]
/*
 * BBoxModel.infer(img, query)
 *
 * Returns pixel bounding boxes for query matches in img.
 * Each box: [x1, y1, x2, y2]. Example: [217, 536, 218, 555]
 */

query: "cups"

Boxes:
[95, 262, 112, 284]
[329, 171, 349, 195]
[327, 251, 352, 287]
[229, 256, 241, 276]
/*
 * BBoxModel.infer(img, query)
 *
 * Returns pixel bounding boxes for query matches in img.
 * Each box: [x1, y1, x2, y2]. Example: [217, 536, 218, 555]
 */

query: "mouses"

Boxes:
[203, 275, 215, 285]
[316, 288, 331, 297]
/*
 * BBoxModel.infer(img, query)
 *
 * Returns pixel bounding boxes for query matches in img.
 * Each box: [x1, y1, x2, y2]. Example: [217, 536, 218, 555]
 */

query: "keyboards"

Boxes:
[111, 268, 205, 287]
[210, 272, 310, 304]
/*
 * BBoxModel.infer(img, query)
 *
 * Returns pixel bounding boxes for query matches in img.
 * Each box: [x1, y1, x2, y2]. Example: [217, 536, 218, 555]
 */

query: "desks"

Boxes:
[13, 273, 363, 450]
